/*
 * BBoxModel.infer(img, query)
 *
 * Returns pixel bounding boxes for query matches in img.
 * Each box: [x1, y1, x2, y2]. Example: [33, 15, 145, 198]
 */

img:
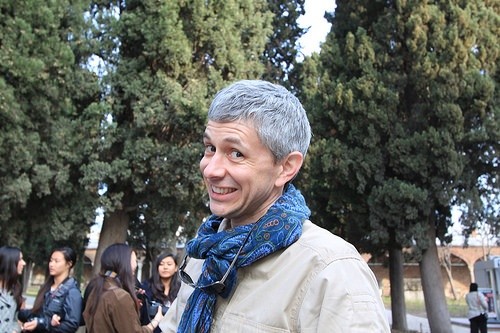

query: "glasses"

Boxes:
[178, 225, 255, 294]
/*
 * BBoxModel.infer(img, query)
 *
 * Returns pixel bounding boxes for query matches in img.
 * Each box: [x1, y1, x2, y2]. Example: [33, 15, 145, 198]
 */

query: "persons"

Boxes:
[0, 245, 27, 333]
[18, 247, 82, 333]
[466, 283, 488, 333]
[144, 253, 196, 333]
[51, 278, 149, 333]
[84, 243, 164, 333]
[158, 80, 391, 333]
